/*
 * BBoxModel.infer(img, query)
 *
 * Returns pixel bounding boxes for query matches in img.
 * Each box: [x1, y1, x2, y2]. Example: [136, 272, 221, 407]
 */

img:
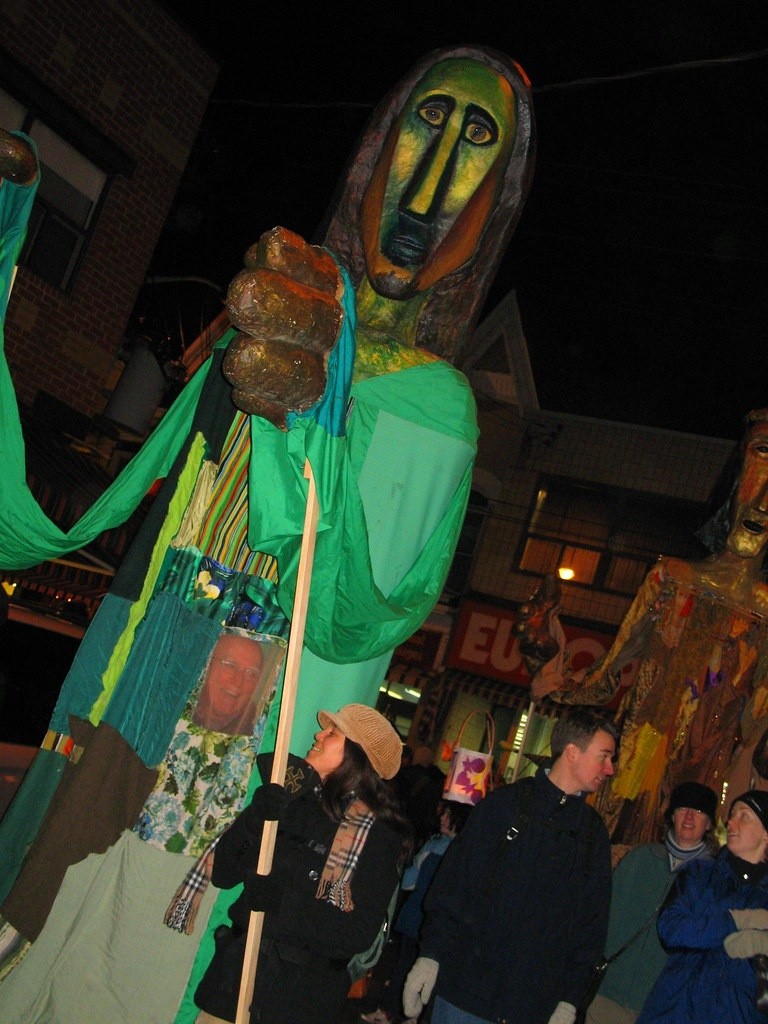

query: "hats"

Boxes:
[725, 790, 768, 834]
[665, 782, 717, 829]
[318, 703, 403, 780]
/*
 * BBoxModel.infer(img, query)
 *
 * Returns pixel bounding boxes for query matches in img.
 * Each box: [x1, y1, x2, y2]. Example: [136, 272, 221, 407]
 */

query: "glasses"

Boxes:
[213, 657, 260, 682]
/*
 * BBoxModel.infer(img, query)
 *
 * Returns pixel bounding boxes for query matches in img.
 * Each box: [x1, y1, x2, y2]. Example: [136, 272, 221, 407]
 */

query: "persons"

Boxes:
[403, 710, 617, 1024]
[193, 704, 405, 1024]
[196, 635, 261, 736]
[517, 407, 768, 843]
[352, 746, 470, 1023]
[634, 789, 768, 1024]
[585, 781, 722, 1024]
[0, 43, 536, 1024]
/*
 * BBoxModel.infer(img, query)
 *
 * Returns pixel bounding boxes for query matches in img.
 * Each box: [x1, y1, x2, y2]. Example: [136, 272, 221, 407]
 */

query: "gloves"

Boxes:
[547, 1001, 577, 1024]
[729, 908, 768, 930]
[244, 869, 285, 913]
[403, 956, 440, 1017]
[246, 784, 289, 837]
[724, 929, 768, 959]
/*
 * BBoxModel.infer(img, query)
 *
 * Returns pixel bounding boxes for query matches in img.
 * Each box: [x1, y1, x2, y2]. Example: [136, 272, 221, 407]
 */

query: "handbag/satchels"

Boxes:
[577, 961, 605, 1018]
[748, 954, 768, 1013]
[442, 709, 495, 805]
